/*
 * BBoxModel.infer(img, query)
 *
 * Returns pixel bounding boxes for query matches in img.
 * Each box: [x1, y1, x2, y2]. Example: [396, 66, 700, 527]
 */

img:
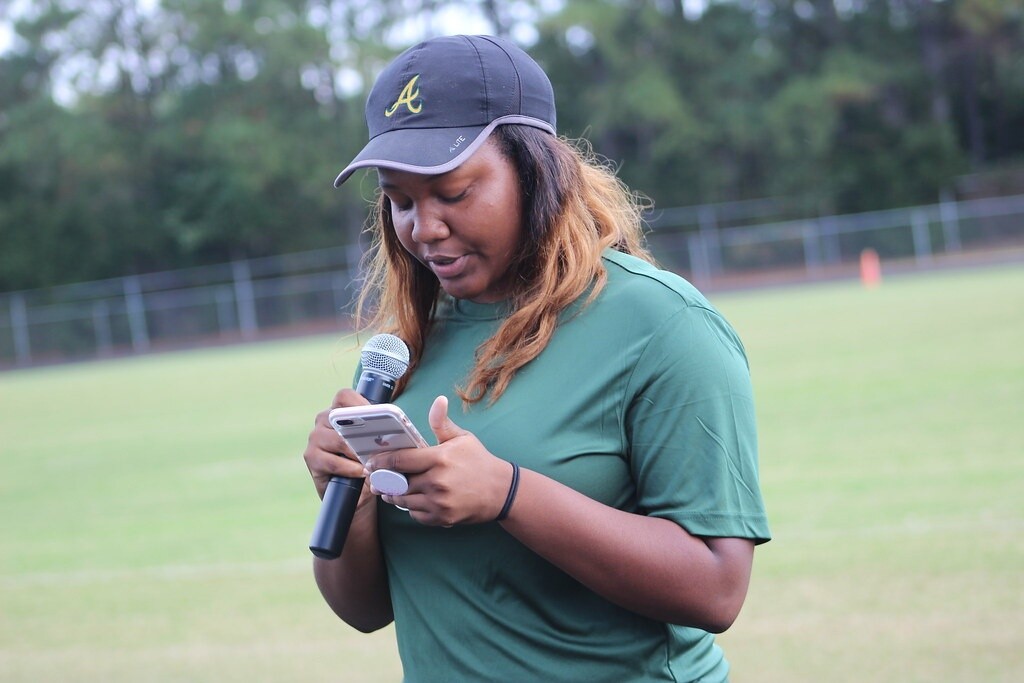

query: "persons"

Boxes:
[304, 28, 771, 683]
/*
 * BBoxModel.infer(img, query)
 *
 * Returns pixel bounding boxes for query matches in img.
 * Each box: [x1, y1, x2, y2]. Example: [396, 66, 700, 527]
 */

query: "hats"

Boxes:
[335, 36, 557, 193]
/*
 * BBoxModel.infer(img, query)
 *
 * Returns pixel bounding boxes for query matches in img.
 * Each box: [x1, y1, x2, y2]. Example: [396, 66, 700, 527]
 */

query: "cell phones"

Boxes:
[327, 403, 429, 512]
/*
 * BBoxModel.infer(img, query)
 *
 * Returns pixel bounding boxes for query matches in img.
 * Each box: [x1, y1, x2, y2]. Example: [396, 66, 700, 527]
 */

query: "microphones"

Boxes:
[309, 335, 409, 560]
